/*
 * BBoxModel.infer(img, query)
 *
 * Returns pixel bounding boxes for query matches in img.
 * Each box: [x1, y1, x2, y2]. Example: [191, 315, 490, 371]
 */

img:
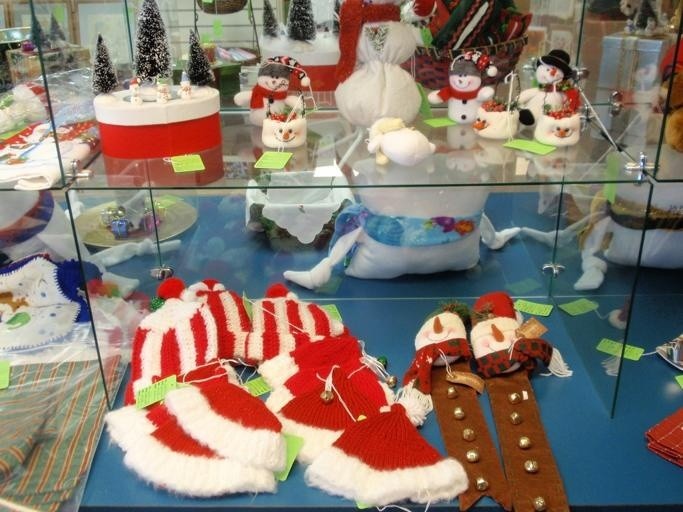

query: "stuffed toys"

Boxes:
[235, 0, 683, 395]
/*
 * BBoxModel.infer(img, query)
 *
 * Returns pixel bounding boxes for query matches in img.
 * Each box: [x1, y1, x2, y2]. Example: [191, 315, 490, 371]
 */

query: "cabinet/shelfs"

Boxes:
[0, 0, 679, 416]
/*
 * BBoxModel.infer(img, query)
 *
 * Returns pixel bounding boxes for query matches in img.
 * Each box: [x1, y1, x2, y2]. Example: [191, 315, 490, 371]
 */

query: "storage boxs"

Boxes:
[593, 31, 674, 95]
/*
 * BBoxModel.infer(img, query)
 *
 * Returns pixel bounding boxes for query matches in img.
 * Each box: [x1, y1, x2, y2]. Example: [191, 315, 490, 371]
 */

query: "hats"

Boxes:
[101, 278, 470, 504]
